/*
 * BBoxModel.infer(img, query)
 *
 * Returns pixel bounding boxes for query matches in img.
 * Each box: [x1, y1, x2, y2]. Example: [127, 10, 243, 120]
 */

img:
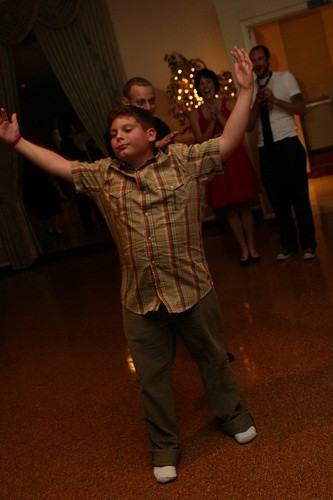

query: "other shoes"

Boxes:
[276, 249, 291, 259]
[303, 246, 317, 258]
[239, 254, 248, 267]
[248, 254, 260, 263]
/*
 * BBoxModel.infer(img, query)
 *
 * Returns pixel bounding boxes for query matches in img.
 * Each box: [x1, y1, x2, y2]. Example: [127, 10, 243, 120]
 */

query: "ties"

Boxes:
[255, 71, 272, 147]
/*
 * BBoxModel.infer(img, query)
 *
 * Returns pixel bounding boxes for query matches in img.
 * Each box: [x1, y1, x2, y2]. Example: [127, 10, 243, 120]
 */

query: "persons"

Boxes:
[0, 46, 257, 482]
[20, 44, 318, 363]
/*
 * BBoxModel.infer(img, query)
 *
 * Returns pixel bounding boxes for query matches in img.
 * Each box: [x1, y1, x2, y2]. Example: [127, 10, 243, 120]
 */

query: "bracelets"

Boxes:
[11, 134, 21, 147]
